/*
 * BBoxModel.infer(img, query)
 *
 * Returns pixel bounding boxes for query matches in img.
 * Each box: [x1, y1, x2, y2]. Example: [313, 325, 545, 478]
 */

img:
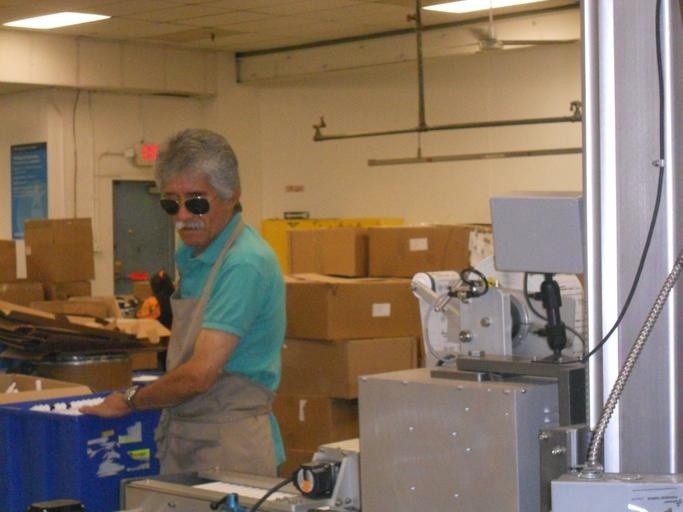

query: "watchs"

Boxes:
[126, 385, 139, 413]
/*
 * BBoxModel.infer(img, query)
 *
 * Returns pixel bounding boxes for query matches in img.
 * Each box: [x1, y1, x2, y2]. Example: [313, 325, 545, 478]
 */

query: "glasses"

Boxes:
[158, 190, 221, 215]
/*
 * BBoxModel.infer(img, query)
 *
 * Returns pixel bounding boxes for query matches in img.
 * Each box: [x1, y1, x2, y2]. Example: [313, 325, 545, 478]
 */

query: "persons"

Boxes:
[79, 128, 287, 477]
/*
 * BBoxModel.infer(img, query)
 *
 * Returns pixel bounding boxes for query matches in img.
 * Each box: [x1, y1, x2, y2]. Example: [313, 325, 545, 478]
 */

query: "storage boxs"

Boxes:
[1, 373, 94, 404]
[276, 338, 417, 400]
[10, 391, 165, 512]
[23, 216, 100, 280]
[285, 228, 368, 277]
[368, 223, 494, 274]
[44, 281, 92, 299]
[2, 280, 44, 305]
[267, 397, 357, 481]
[281, 279, 423, 340]
[0, 240, 17, 282]
